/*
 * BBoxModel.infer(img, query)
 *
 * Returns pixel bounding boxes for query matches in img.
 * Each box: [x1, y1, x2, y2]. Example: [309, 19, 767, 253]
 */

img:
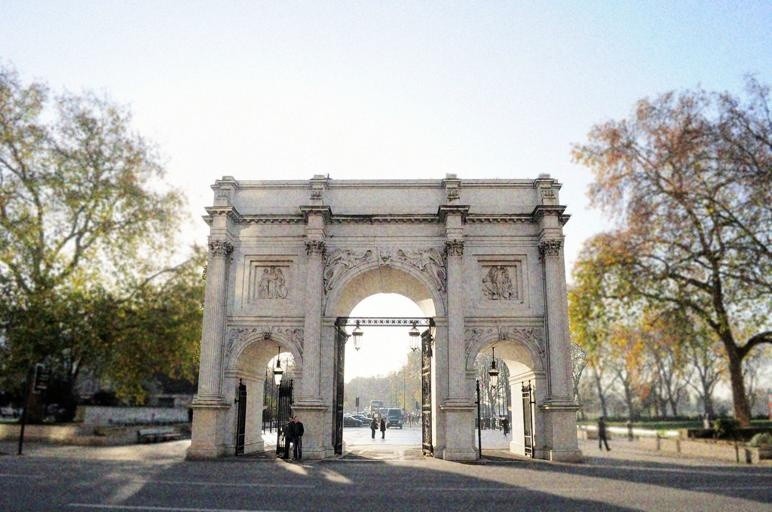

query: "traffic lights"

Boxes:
[36, 366, 52, 393]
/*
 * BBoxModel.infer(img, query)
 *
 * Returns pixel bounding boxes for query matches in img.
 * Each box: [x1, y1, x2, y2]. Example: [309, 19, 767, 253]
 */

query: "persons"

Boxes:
[261, 266, 286, 299]
[281, 417, 295, 459]
[370, 418, 379, 438]
[598, 417, 611, 451]
[500, 417, 508, 436]
[482, 266, 513, 300]
[293, 416, 304, 460]
[380, 419, 386, 439]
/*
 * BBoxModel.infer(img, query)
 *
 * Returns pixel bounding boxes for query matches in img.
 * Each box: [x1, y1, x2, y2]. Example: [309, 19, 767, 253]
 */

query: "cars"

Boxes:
[343, 400, 404, 428]
